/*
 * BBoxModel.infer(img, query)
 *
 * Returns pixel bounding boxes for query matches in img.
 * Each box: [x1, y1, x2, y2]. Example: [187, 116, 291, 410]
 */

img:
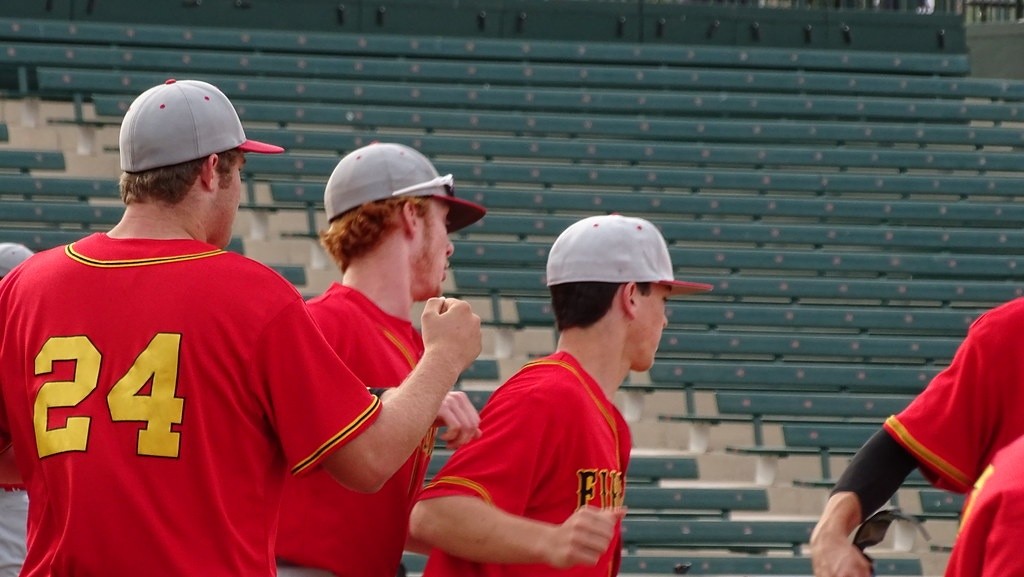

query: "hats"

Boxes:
[118, 77, 283, 174]
[325, 140, 487, 234]
[0, 242, 35, 277]
[546, 211, 714, 294]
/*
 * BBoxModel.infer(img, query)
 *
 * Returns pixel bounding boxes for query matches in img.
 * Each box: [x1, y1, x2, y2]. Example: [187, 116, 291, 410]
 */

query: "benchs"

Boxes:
[0, 0, 1024, 577]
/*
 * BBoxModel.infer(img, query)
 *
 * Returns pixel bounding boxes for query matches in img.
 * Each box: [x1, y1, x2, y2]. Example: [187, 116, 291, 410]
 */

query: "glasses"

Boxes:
[391, 172, 455, 198]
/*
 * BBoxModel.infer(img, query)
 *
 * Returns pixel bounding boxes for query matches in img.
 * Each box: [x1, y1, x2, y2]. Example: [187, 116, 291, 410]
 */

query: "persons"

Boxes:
[809, 295, 1024, 577]
[945, 436, 1024, 577]
[266, 138, 488, 577]
[0, 243, 36, 577]
[413, 215, 714, 577]
[0, 76, 484, 577]
